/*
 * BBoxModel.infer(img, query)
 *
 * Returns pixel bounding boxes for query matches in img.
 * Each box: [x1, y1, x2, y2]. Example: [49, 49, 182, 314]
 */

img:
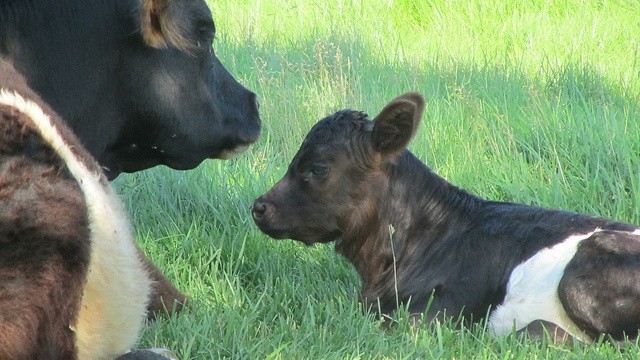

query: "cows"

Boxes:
[252, 90, 640, 343]
[1, 0, 264, 360]
